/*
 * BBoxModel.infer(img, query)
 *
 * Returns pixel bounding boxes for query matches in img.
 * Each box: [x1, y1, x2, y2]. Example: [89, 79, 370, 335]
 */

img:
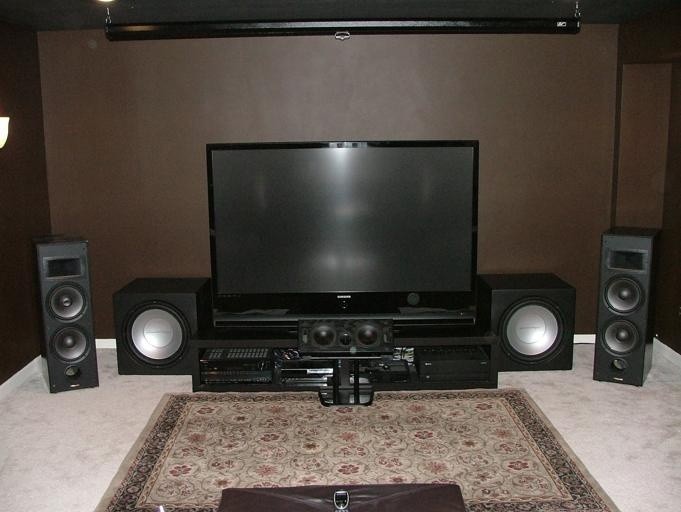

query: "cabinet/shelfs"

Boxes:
[188, 323, 500, 391]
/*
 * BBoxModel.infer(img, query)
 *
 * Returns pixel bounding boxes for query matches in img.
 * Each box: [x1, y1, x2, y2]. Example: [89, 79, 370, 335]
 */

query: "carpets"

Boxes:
[93, 386, 621, 512]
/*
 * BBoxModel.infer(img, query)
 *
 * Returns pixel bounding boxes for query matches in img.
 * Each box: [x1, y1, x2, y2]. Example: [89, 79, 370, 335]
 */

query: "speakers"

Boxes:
[592, 226, 662, 388]
[31, 231, 99, 394]
[111, 277, 211, 377]
[479, 273, 578, 372]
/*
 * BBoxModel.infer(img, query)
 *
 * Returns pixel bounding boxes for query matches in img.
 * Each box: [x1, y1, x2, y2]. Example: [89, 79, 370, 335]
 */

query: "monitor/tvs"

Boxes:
[205, 139, 480, 318]
